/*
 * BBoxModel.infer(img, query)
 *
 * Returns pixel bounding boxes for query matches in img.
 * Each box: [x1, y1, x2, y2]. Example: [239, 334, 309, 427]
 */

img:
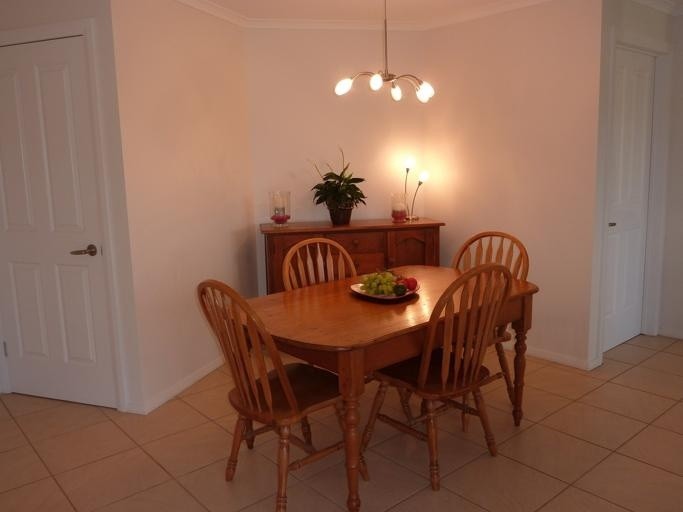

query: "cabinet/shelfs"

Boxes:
[260, 217, 446, 295]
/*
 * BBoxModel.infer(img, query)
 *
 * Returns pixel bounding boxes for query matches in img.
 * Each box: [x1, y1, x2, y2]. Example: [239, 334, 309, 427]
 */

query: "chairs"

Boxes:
[361, 263, 512, 491]
[282, 238, 416, 429]
[450, 232, 529, 432]
[197, 279, 370, 512]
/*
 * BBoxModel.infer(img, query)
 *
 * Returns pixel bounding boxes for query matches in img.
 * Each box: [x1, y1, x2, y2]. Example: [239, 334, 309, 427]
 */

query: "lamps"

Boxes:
[405, 156, 427, 220]
[335, 0, 434, 103]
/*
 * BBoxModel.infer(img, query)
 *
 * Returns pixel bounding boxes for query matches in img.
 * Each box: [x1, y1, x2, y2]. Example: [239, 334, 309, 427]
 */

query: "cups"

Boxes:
[387, 192, 408, 224]
[265, 190, 291, 228]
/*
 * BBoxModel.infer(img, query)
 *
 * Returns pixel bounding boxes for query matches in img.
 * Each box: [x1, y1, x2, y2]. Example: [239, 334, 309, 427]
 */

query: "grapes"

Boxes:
[360, 269, 402, 296]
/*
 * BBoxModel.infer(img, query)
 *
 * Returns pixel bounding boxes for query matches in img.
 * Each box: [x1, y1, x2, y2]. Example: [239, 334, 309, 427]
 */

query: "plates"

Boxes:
[348, 281, 420, 302]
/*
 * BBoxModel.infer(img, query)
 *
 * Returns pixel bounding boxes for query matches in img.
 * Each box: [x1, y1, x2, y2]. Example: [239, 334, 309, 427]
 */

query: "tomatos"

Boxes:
[404, 278, 417, 290]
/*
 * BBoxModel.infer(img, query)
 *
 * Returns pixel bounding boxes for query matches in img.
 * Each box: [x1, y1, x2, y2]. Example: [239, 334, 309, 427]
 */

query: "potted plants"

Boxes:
[310, 145, 367, 225]
